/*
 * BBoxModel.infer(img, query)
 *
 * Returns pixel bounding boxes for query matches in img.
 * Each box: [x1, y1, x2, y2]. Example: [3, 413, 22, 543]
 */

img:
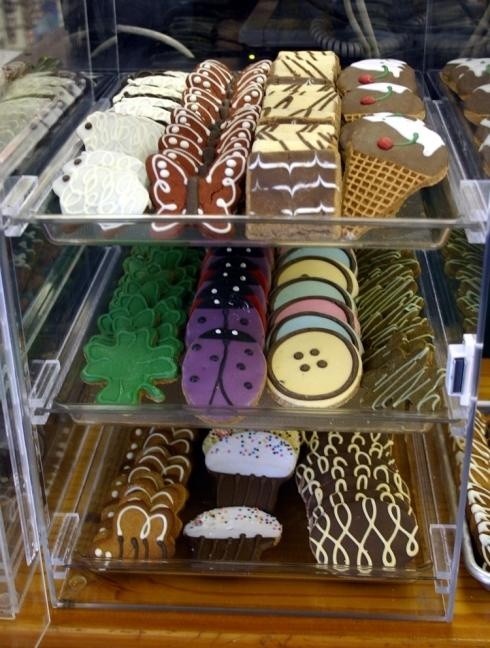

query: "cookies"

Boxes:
[79, 241, 447, 415]
[85, 421, 423, 577]
[0, 49, 490, 240]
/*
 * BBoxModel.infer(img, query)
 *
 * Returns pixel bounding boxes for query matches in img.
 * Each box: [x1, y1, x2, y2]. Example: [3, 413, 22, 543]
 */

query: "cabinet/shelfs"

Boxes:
[0, 1, 490, 620]
